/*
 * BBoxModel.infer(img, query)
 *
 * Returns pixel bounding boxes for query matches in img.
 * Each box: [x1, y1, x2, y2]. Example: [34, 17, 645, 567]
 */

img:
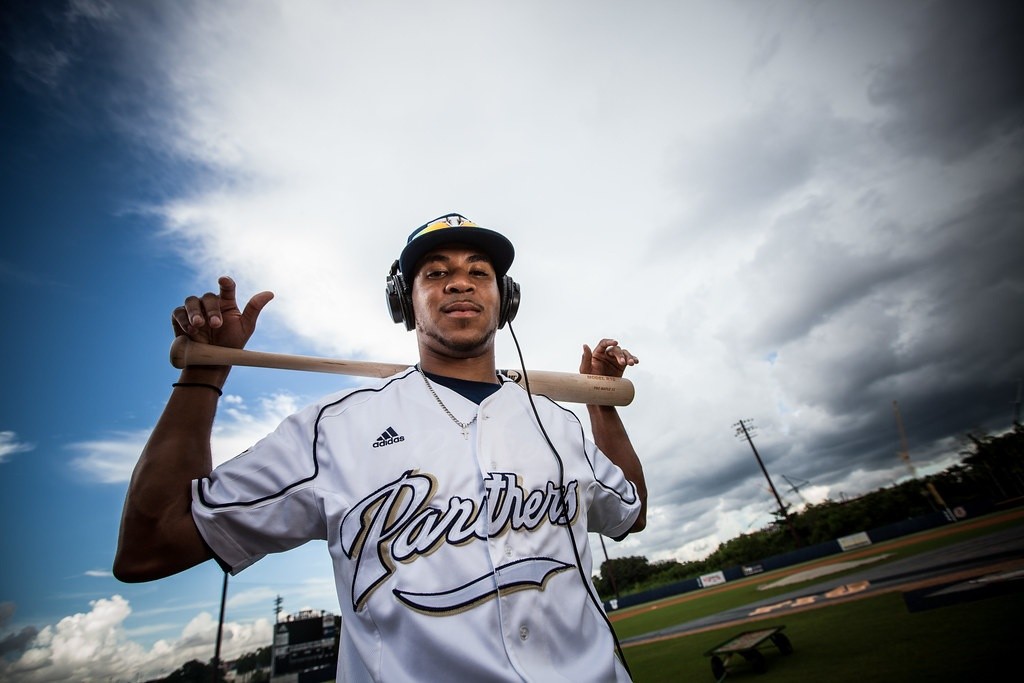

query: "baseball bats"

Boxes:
[169, 335, 634, 406]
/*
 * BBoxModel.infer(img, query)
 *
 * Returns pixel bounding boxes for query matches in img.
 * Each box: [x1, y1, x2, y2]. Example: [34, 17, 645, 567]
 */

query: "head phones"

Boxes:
[385, 259, 520, 333]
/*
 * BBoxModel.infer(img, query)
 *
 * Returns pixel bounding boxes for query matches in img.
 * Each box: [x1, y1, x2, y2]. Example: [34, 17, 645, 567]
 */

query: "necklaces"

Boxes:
[418, 361, 478, 440]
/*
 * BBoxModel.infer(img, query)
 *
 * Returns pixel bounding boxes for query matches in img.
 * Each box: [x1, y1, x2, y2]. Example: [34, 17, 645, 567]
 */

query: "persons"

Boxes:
[113, 213, 648, 683]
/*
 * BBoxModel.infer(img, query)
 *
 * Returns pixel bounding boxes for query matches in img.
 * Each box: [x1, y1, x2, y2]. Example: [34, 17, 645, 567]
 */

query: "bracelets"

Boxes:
[171, 384, 223, 397]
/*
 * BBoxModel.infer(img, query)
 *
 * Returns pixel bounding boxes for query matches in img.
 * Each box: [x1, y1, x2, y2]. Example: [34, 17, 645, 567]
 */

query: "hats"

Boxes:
[398, 213, 515, 277]
